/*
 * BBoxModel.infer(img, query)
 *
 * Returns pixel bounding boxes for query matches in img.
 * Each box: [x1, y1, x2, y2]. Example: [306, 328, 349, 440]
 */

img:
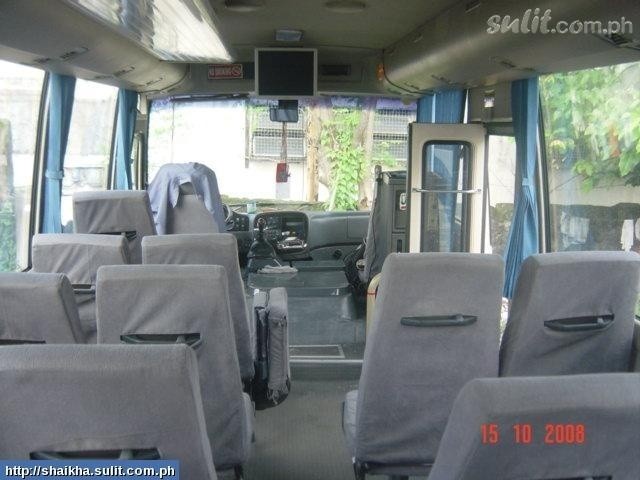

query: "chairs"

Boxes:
[345, 171, 440, 304]
[342, 251, 507, 480]
[146, 163, 223, 233]
[427, 371, 640, 480]
[0, 342, 217, 480]
[71, 190, 156, 265]
[96, 265, 258, 480]
[139, 234, 255, 379]
[0, 272, 89, 341]
[500, 250, 640, 377]
[28, 233, 128, 343]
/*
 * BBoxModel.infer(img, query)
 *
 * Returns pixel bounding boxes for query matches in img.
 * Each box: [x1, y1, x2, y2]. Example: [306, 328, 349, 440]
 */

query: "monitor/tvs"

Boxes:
[255, 47, 318, 100]
